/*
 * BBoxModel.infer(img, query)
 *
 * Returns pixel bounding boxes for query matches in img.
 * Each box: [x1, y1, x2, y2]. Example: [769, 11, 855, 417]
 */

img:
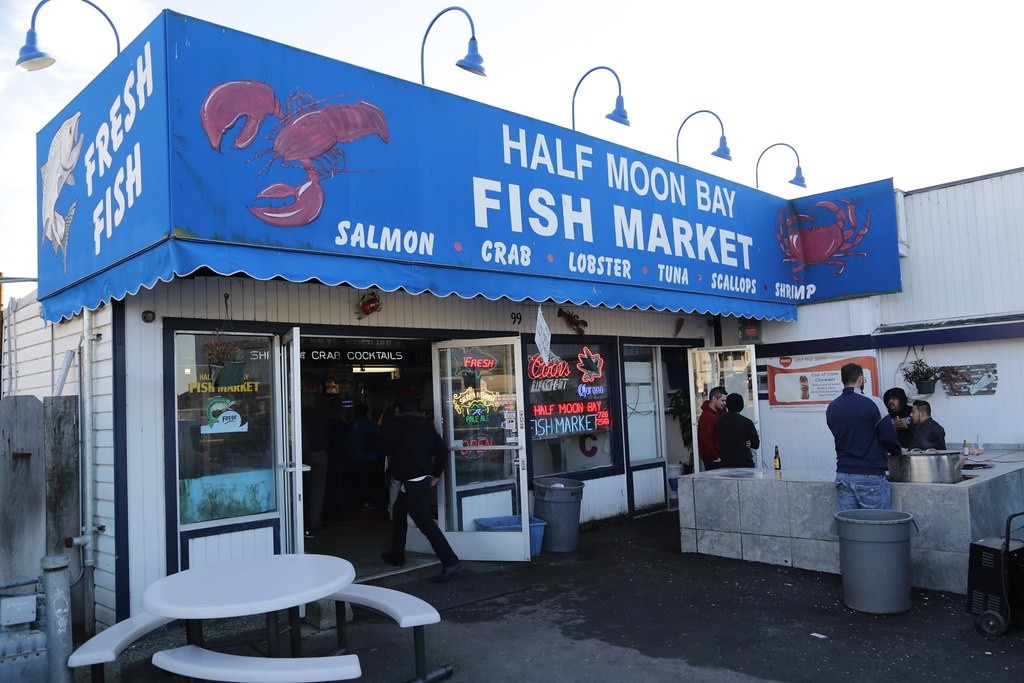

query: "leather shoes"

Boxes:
[381, 551, 405, 566]
[431, 561, 464, 583]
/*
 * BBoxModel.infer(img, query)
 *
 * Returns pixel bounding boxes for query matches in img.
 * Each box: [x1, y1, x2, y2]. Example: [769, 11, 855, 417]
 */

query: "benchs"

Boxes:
[324, 583, 454, 683]
[152, 644, 362, 683]
[68, 610, 178, 682]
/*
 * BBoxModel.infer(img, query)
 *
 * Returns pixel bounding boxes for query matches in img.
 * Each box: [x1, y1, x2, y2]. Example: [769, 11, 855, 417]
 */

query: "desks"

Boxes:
[141, 554, 356, 683]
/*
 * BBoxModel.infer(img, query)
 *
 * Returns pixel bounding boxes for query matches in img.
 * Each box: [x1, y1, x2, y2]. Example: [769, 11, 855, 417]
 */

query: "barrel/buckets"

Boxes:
[668, 465, 683, 499]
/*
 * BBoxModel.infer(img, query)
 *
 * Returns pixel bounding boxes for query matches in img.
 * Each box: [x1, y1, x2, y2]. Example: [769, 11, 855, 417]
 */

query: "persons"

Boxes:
[908, 400, 946, 450]
[825, 360, 901, 576]
[716, 393, 761, 469]
[697, 386, 728, 471]
[300, 393, 382, 539]
[374, 397, 466, 582]
[882, 387, 916, 447]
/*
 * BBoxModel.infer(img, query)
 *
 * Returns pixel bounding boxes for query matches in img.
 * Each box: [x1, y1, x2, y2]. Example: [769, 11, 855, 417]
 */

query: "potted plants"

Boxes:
[901, 359, 943, 395]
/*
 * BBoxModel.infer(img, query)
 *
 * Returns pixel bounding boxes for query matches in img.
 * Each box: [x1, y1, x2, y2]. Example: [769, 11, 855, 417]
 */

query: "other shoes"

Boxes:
[306, 523, 327, 531]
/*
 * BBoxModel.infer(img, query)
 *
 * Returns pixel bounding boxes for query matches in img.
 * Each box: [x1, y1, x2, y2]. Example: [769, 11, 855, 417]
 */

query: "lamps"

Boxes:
[421, 6, 487, 85]
[676, 110, 731, 163]
[572, 66, 630, 131]
[15, 0, 120, 72]
[756, 143, 807, 189]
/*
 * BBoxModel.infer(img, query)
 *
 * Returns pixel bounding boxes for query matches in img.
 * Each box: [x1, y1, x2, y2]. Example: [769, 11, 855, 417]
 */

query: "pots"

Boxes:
[887, 448, 968, 484]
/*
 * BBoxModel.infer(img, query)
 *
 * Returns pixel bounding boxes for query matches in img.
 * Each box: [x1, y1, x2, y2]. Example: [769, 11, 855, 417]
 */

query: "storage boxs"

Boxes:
[473, 515, 547, 557]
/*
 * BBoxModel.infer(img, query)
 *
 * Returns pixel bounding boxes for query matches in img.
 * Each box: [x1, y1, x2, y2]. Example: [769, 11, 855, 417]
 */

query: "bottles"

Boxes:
[963, 435, 984, 456]
[773, 446, 782, 471]
[799, 374, 809, 400]
